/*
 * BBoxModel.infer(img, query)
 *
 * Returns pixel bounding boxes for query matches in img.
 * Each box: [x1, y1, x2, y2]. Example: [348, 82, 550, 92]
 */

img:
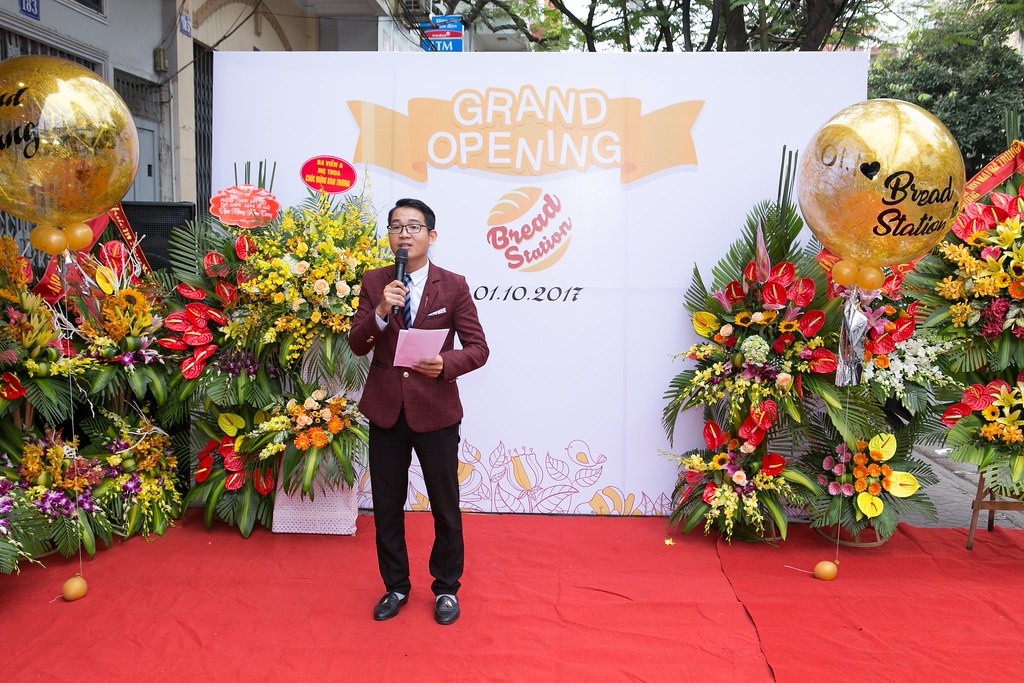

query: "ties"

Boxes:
[399, 275, 412, 329]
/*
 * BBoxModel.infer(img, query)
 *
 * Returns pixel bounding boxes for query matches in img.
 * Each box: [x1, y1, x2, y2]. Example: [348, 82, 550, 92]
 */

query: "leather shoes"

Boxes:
[372, 591, 408, 620]
[435, 593, 460, 624]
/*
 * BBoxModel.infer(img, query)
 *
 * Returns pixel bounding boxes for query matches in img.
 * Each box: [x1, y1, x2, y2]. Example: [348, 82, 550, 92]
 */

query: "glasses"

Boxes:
[386, 223, 432, 234]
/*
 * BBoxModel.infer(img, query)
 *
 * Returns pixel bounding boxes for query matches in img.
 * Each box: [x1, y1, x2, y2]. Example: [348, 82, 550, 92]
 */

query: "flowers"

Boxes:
[662, 108, 1024, 546]
[0, 160, 398, 575]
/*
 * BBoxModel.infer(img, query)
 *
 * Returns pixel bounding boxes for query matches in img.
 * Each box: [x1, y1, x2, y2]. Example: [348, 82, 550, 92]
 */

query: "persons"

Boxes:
[349, 199, 491, 624]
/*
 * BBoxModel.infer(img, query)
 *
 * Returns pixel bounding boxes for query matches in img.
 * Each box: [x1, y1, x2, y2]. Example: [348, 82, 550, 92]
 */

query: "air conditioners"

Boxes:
[400, 0, 432, 24]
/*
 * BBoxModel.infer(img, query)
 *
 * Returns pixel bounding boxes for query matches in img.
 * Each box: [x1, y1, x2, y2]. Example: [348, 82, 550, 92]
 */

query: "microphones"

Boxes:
[391, 248, 408, 314]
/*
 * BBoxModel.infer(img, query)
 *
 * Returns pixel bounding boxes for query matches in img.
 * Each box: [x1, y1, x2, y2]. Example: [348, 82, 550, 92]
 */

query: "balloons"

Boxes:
[857, 266, 885, 290]
[832, 261, 858, 285]
[29, 223, 93, 256]
[0, 55, 139, 229]
[798, 99, 966, 266]
